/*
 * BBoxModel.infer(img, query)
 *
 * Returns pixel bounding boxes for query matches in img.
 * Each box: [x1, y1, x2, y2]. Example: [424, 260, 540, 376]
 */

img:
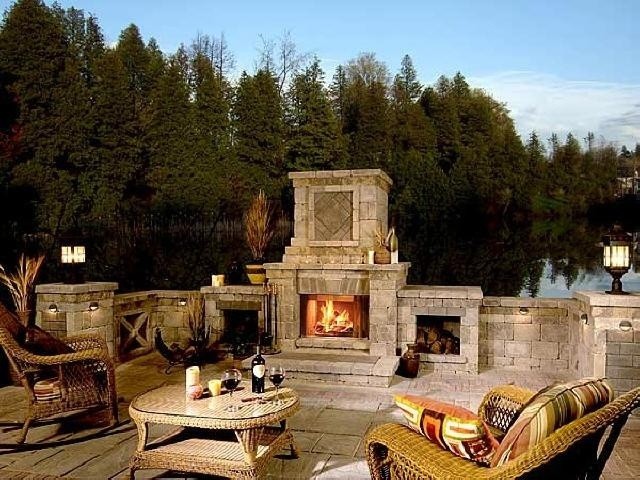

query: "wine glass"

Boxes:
[268, 365, 284, 406]
[222, 369, 243, 413]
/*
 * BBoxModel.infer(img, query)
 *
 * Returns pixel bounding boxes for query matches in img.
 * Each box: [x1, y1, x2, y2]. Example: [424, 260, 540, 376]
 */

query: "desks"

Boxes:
[120, 378, 302, 480]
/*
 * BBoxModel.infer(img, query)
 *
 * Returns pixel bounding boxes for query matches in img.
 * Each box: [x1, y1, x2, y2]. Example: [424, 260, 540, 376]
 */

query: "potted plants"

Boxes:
[0, 252, 44, 327]
[245, 189, 274, 286]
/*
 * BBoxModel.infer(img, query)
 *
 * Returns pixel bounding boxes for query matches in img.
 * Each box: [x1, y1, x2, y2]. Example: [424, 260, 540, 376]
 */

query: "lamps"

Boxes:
[57, 227, 89, 284]
[600, 224, 633, 295]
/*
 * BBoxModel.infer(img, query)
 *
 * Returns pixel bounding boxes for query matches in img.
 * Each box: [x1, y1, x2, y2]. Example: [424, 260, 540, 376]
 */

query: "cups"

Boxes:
[186, 366, 200, 392]
[208, 380, 221, 396]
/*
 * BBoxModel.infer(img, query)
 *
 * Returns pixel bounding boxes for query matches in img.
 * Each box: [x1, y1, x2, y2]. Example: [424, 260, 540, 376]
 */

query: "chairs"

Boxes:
[0, 305, 120, 444]
[362, 384, 640, 479]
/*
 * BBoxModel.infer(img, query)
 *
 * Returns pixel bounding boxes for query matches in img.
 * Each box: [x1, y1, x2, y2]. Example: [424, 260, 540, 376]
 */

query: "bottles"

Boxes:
[368, 246, 375, 265]
[251, 344, 266, 393]
[390, 226, 399, 264]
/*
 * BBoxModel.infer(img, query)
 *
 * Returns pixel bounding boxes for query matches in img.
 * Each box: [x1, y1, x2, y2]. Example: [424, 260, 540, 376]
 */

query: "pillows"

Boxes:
[390, 392, 500, 467]
[25, 325, 77, 354]
[490, 376, 616, 469]
